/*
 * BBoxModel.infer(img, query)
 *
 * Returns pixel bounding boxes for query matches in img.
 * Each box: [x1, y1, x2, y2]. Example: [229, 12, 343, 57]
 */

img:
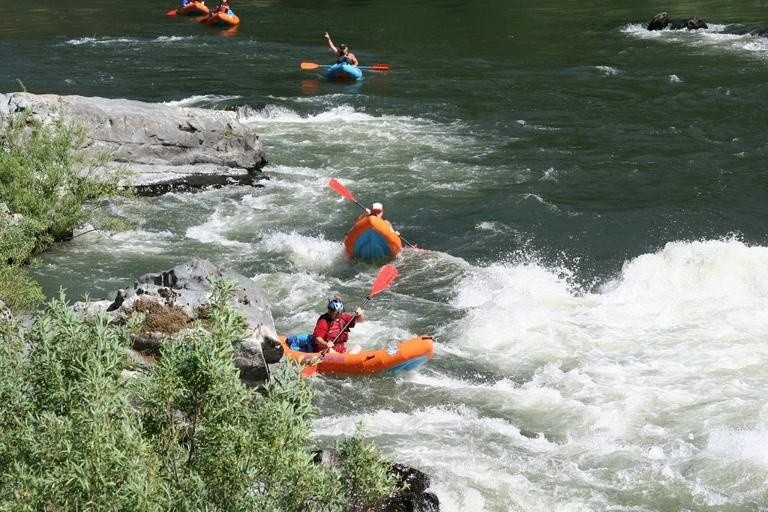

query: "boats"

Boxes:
[204, 24, 240, 37]
[199, 12, 241, 25]
[176, 2, 209, 16]
[326, 63, 363, 82]
[278, 334, 435, 376]
[344, 212, 403, 263]
[330, 83, 363, 94]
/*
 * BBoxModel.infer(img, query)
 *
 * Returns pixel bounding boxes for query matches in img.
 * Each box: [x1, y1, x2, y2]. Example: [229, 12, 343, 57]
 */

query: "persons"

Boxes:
[214, 0, 229, 13]
[325, 32, 358, 66]
[313, 300, 364, 354]
[358, 202, 400, 237]
[183, 0, 192, 7]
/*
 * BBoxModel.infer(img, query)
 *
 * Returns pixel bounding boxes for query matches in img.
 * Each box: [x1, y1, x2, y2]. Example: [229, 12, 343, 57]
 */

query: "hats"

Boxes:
[371, 202, 385, 211]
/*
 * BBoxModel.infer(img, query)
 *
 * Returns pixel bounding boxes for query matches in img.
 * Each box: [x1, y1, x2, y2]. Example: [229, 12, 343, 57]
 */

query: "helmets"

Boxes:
[327, 299, 345, 314]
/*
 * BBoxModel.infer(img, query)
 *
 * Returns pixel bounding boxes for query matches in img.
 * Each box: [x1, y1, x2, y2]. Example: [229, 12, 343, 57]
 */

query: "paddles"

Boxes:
[164, 6, 184, 15]
[329, 178, 423, 251]
[301, 62, 389, 72]
[301, 263, 398, 377]
